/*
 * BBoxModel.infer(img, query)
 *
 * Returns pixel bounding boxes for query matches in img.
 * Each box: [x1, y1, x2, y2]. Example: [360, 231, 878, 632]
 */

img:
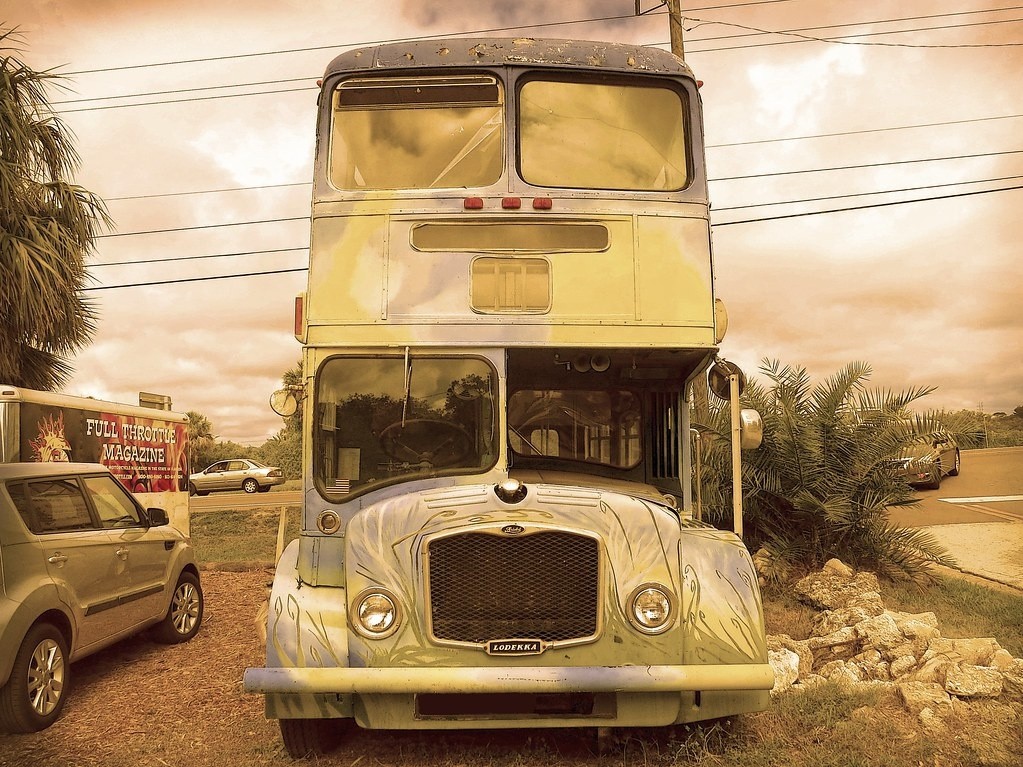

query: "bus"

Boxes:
[236, 35, 776, 759]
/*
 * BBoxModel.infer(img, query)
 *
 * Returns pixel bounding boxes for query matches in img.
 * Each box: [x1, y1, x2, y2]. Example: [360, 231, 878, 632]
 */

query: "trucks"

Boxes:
[0, 383, 194, 541]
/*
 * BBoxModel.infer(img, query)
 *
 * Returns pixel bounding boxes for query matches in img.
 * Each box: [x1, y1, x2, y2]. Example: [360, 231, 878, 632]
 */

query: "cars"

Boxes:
[873, 418, 962, 490]
[188, 457, 286, 497]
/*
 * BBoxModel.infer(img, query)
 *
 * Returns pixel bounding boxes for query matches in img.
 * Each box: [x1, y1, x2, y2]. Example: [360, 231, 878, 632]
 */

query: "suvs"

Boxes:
[0, 461, 204, 735]
